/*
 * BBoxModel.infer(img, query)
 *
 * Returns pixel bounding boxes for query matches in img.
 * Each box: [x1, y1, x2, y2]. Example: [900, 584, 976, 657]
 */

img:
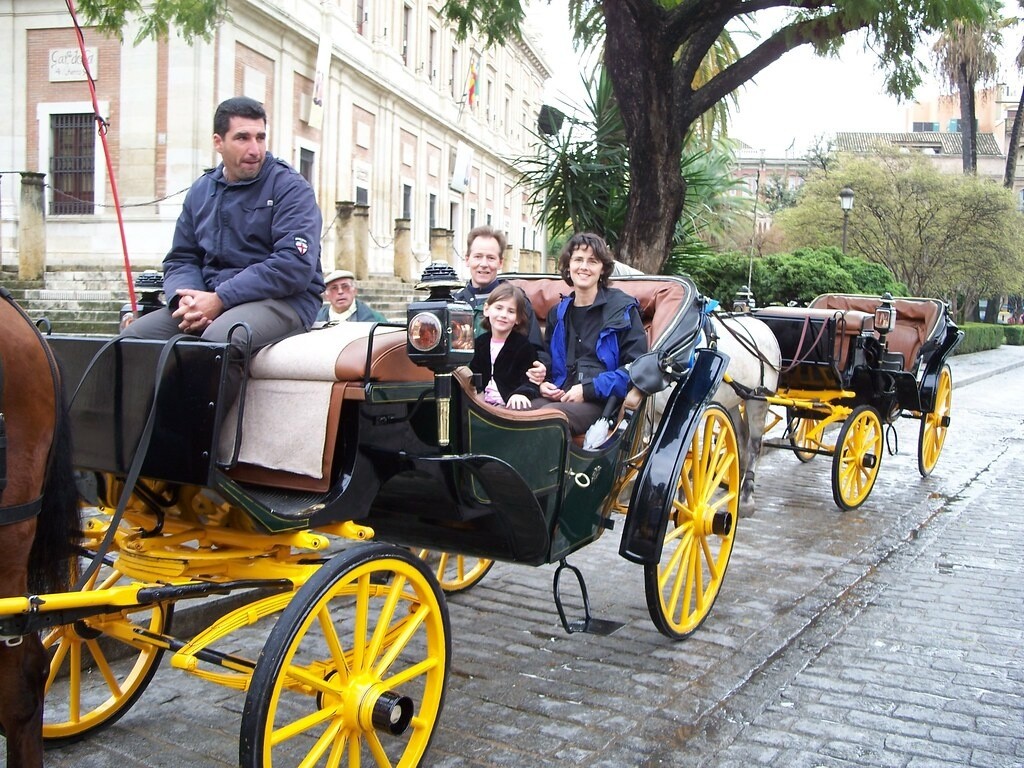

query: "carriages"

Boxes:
[0, 269, 741, 768]
[610, 256, 964, 511]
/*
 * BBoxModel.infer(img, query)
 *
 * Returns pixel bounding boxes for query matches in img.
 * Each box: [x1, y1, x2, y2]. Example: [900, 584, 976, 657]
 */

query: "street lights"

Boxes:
[838, 187, 856, 253]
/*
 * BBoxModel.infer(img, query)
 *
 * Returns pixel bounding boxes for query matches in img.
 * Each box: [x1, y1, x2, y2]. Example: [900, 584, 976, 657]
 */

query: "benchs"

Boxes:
[886, 324, 922, 372]
[758, 307, 876, 336]
[249, 321, 436, 405]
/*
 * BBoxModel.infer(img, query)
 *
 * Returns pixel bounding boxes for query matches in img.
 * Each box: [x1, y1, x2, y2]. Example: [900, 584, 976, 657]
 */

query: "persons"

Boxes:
[506, 232, 649, 438]
[315, 270, 389, 322]
[119, 97, 326, 515]
[452, 224, 552, 410]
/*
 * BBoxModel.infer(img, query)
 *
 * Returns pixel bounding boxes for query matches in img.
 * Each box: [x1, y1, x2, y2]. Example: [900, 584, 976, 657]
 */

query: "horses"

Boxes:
[607, 243, 783, 520]
[0, 286, 85, 768]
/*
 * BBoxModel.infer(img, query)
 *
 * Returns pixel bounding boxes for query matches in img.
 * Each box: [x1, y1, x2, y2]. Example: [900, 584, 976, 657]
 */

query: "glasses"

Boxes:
[327, 283, 354, 292]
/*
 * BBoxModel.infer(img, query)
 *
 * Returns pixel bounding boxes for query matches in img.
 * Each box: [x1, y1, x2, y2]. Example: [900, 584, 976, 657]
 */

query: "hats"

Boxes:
[323, 269, 355, 284]
